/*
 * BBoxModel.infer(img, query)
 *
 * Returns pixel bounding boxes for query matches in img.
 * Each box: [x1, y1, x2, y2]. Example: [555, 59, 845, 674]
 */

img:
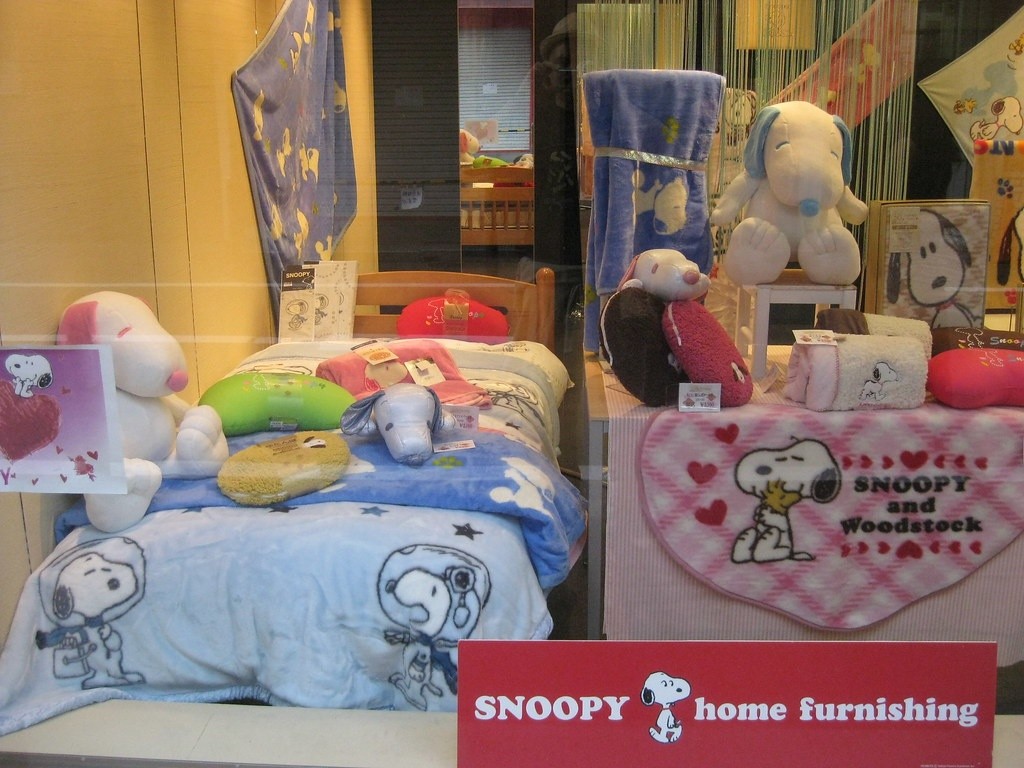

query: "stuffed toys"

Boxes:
[709, 100, 874, 286]
[53, 289, 231, 537]
[616, 247, 712, 305]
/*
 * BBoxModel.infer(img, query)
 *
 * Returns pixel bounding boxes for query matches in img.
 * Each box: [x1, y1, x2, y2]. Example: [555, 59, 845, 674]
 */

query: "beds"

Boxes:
[0, 330, 574, 739]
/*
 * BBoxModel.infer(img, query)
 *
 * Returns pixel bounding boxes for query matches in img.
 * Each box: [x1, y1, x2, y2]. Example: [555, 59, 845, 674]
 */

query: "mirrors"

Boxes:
[457, 0, 537, 287]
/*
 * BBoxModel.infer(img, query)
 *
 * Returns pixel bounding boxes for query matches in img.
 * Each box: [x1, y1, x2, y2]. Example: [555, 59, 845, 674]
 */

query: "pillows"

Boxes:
[929, 319, 1023, 357]
[398, 292, 512, 342]
[926, 345, 1024, 409]
[473, 155, 510, 168]
[196, 368, 357, 432]
